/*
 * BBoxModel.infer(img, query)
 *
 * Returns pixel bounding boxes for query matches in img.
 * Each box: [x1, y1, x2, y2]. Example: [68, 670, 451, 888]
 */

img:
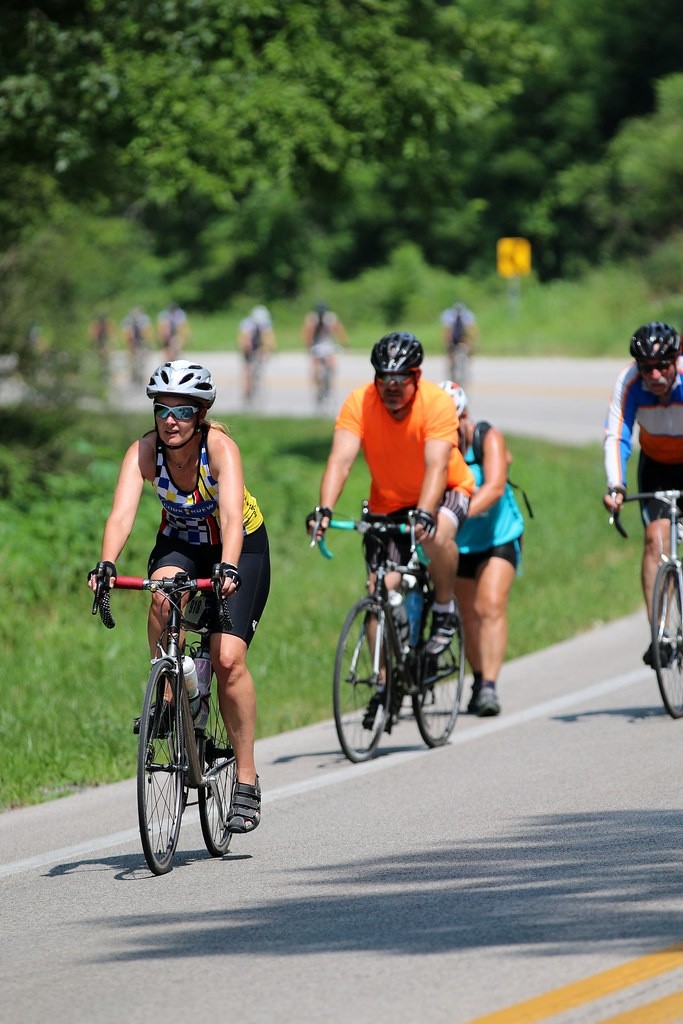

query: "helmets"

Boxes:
[631, 322, 683, 361]
[147, 360, 215, 403]
[371, 331, 423, 374]
[439, 380, 467, 417]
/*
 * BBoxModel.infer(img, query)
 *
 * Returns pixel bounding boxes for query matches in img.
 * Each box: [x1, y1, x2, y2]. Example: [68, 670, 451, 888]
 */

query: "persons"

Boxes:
[238, 305, 274, 396]
[155, 302, 189, 359]
[438, 379, 525, 717]
[441, 303, 478, 361]
[603, 323, 683, 668]
[87, 360, 272, 832]
[302, 302, 348, 395]
[90, 314, 120, 360]
[304, 331, 474, 732]
[122, 307, 153, 361]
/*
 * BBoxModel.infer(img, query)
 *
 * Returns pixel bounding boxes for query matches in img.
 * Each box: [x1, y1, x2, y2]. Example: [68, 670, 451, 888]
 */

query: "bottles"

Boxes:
[389, 591, 411, 654]
[405, 582, 422, 646]
[188, 652, 213, 730]
[181, 654, 203, 725]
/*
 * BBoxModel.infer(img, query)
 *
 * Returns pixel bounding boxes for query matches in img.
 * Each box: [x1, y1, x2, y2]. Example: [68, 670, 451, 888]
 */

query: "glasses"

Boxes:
[639, 358, 675, 372]
[376, 369, 412, 385]
[154, 402, 200, 421]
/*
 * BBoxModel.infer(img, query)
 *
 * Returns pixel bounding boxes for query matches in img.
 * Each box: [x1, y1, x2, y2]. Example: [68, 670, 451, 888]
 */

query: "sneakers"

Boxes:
[226, 776, 261, 833]
[134, 701, 175, 740]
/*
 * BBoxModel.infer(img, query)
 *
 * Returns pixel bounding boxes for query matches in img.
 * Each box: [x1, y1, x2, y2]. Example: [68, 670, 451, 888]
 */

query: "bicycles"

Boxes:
[92, 560, 241, 877]
[310, 505, 467, 763]
[605, 484, 683, 722]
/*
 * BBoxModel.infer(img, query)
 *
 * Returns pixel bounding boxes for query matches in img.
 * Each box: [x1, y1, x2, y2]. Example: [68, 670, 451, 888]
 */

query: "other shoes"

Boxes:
[643, 638, 673, 668]
[468, 689, 501, 717]
[425, 619, 457, 655]
[363, 698, 393, 734]
